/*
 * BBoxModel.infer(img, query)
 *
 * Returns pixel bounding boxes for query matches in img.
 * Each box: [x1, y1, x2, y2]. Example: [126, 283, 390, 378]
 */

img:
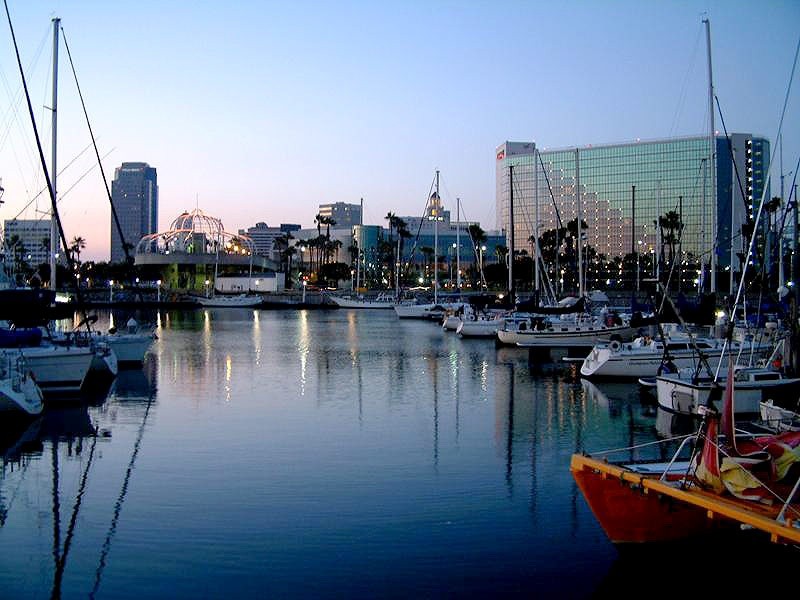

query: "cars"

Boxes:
[440, 278, 450, 288]
[448, 278, 466, 288]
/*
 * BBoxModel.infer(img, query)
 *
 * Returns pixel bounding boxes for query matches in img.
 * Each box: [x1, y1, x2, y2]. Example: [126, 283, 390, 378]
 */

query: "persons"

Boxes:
[772, 348, 782, 368]
[644, 336, 656, 346]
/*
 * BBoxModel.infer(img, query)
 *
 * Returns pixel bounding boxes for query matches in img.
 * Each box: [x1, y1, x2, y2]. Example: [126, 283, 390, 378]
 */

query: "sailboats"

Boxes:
[189, 244, 265, 308]
[0, 0, 163, 427]
[320, 9, 800, 600]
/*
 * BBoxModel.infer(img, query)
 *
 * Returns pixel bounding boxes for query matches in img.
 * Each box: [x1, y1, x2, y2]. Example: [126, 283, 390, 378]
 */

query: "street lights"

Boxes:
[450, 243, 457, 285]
[534, 224, 545, 291]
[479, 245, 488, 292]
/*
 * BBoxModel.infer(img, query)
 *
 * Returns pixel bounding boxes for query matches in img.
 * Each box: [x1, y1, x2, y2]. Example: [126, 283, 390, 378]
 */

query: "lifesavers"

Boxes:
[610, 340, 621, 351]
[469, 314, 475, 320]
